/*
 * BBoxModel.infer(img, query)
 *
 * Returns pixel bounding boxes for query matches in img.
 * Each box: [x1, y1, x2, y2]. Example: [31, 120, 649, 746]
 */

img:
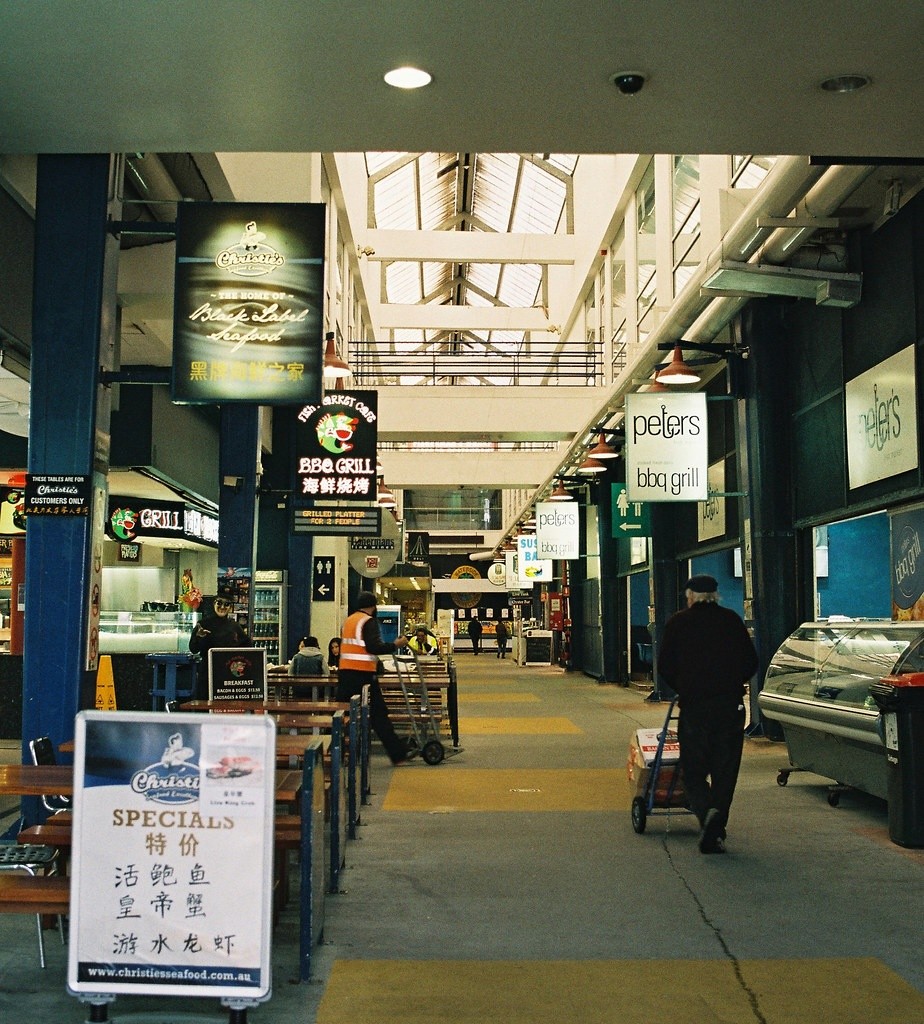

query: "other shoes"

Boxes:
[699, 808, 722, 852]
[393, 749, 420, 767]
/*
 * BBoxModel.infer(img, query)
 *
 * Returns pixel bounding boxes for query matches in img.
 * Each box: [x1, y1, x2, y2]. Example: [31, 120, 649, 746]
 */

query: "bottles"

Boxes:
[253, 591, 279, 665]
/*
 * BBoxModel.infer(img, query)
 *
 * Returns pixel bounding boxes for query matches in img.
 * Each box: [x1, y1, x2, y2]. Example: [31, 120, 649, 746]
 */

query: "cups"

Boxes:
[139, 601, 178, 612]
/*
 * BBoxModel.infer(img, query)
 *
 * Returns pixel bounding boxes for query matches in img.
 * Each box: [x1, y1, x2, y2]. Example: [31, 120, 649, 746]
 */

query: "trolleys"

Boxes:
[631, 695, 694, 834]
[392, 637, 466, 765]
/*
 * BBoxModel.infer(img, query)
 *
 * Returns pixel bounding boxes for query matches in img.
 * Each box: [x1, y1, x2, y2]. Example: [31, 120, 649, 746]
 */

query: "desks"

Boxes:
[0, 648, 459, 981]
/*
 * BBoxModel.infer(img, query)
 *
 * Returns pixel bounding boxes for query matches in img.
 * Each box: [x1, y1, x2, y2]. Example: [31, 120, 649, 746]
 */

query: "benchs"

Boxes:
[384, 704, 443, 714]
[386, 713, 443, 737]
[45, 810, 302, 829]
[17, 827, 304, 876]
[1, 876, 68, 915]
[382, 697, 442, 705]
[383, 690, 442, 699]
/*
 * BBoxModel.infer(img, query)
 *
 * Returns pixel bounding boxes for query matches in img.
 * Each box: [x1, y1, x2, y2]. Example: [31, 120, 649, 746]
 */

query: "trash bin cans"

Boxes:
[868, 672, 924, 849]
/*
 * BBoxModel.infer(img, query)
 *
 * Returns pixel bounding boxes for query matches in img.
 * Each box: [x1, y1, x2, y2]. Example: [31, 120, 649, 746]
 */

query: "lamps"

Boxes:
[550, 474, 596, 500]
[653, 337, 747, 386]
[322, 332, 352, 378]
[577, 439, 626, 474]
[586, 427, 625, 459]
[643, 356, 722, 393]
[492, 497, 563, 562]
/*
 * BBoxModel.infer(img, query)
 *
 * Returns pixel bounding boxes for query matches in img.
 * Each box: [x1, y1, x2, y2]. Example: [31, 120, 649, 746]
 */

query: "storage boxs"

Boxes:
[627, 728, 688, 802]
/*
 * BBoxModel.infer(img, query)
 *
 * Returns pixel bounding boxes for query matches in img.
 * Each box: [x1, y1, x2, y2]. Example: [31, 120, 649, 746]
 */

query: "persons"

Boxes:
[467, 616, 483, 656]
[405, 628, 437, 655]
[327, 637, 341, 667]
[337, 590, 421, 763]
[286, 635, 330, 699]
[495, 617, 511, 659]
[187, 585, 251, 701]
[656, 574, 756, 852]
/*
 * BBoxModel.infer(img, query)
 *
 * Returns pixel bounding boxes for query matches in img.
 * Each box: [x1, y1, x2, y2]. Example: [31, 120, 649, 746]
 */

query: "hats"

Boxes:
[359, 592, 377, 608]
[683, 575, 718, 593]
[217, 585, 234, 601]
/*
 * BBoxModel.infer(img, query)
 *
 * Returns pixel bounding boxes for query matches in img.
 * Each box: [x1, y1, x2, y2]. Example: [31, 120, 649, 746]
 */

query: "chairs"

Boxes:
[0, 844, 62, 968]
[26, 735, 72, 812]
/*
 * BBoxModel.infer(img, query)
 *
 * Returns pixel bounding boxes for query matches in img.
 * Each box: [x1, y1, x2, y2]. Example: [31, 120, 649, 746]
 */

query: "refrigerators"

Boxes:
[253, 570, 289, 700]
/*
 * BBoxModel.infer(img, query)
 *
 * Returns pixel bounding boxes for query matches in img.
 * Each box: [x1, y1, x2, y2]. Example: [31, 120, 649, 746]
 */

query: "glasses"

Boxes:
[215, 599, 232, 607]
